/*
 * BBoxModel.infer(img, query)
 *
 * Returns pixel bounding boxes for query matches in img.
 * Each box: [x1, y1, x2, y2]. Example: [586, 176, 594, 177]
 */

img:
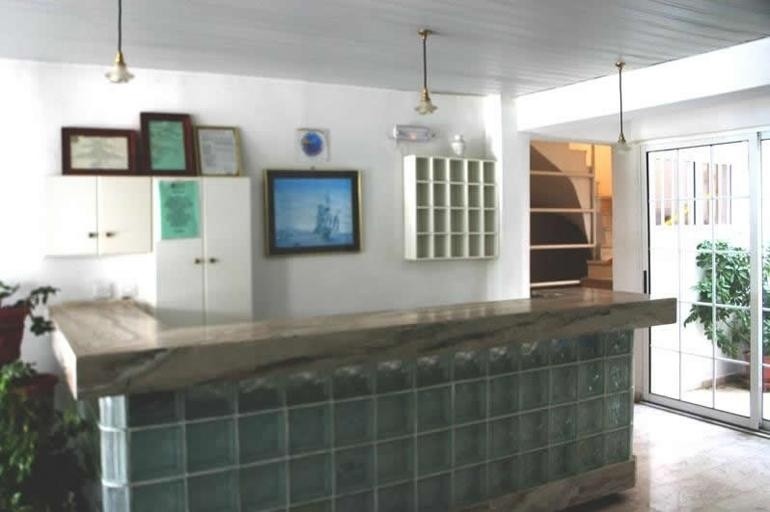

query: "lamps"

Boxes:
[106, 0, 134, 83]
[413, 28, 440, 115]
[613, 61, 631, 153]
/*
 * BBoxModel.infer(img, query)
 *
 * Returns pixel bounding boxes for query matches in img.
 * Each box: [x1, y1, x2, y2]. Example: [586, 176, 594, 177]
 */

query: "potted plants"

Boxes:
[0, 281, 93, 512]
[687, 237, 770, 392]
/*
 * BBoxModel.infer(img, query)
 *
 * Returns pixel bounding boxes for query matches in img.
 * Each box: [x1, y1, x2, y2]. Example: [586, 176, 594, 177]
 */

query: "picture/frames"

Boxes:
[60, 111, 242, 176]
[263, 167, 362, 259]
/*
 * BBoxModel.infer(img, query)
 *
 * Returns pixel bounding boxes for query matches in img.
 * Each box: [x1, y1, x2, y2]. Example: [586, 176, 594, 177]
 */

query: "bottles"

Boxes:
[450, 134, 467, 158]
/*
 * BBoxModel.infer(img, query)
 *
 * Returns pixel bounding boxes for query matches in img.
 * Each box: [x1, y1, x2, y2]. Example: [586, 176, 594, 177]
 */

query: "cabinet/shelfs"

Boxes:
[43, 176, 151, 260]
[134, 175, 252, 329]
[402, 153, 499, 261]
